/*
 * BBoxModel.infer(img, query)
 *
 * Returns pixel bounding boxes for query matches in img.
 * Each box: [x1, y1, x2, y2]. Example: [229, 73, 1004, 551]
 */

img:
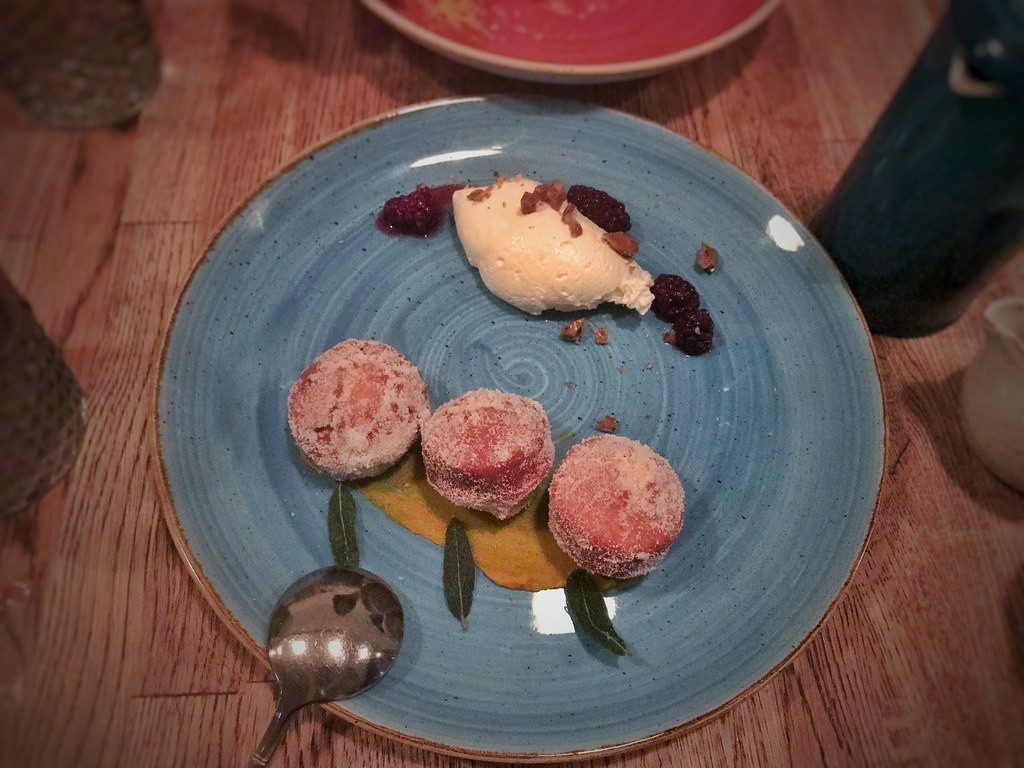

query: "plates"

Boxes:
[149, 94, 889, 765]
[358, 1, 780, 84]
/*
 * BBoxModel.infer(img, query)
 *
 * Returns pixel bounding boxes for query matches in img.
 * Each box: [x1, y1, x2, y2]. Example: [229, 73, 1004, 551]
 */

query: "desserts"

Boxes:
[287, 338, 429, 487]
[418, 386, 557, 519]
[550, 434, 686, 582]
[452, 174, 656, 321]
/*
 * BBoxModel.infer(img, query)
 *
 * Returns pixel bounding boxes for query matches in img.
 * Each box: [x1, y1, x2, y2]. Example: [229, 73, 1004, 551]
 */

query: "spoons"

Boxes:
[245, 563, 404, 767]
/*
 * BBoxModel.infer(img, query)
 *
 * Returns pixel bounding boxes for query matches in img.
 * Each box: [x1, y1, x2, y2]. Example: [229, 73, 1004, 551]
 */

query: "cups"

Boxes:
[954, 300, 1024, 492]
